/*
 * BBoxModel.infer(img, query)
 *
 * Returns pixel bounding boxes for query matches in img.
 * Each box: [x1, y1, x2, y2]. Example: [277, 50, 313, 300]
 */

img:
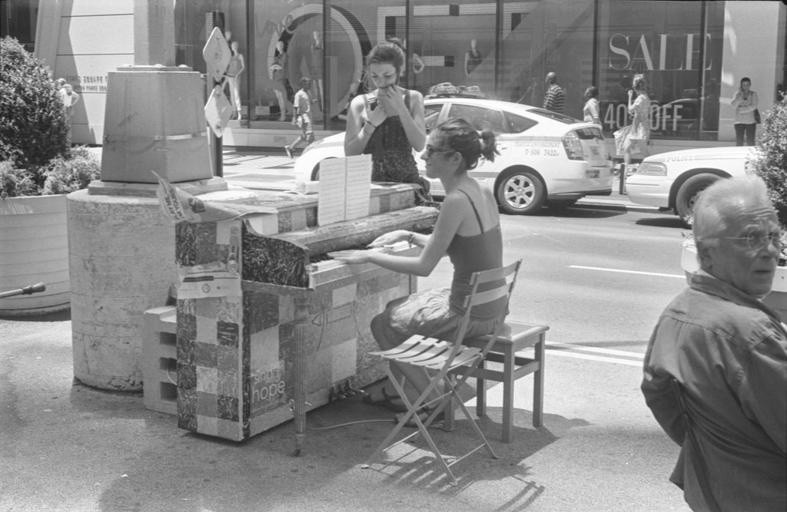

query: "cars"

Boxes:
[294, 82, 611, 212]
[624, 143, 768, 229]
[619, 89, 765, 141]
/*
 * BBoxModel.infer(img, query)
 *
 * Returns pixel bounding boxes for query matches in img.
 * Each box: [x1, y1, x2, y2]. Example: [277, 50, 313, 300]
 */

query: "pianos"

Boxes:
[173, 179, 445, 444]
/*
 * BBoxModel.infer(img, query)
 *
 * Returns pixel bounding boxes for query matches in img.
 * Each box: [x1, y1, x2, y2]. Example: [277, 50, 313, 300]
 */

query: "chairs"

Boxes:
[361, 258, 521, 486]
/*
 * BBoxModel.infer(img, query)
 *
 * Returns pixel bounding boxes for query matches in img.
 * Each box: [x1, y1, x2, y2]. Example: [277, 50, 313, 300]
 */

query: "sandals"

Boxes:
[363, 388, 407, 412]
[394, 402, 445, 427]
[724, 230, 787, 251]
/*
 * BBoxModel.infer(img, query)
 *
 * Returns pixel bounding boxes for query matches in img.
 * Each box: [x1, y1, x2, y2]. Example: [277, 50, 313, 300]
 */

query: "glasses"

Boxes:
[427, 144, 449, 158]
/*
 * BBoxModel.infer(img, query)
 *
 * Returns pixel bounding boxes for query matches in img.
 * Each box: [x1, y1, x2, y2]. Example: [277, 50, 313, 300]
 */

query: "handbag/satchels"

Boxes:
[755, 110, 761, 125]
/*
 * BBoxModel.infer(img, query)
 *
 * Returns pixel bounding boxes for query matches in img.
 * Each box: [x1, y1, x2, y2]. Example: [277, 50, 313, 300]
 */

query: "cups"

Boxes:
[368, 98, 377, 111]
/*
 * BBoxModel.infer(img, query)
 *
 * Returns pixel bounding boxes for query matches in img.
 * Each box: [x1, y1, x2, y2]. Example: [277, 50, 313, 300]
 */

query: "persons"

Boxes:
[341, 36, 428, 202]
[465, 36, 483, 79]
[641, 174, 787, 511]
[581, 84, 602, 126]
[612, 72, 652, 192]
[412, 53, 425, 89]
[226, 40, 246, 120]
[730, 77, 759, 146]
[53, 77, 79, 147]
[541, 70, 566, 115]
[310, 30, 324, 112]
[326, 118, 510, 430]
[284, 76, 315, 160]
[269, 39, 289, 122]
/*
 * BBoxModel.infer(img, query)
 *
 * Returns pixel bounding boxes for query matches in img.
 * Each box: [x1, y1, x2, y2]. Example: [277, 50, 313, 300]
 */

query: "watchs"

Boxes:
[407, 232, 415, 244]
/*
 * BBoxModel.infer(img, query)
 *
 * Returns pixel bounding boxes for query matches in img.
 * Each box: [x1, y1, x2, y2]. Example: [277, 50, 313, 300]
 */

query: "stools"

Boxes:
[463, 322, 551, 445]
